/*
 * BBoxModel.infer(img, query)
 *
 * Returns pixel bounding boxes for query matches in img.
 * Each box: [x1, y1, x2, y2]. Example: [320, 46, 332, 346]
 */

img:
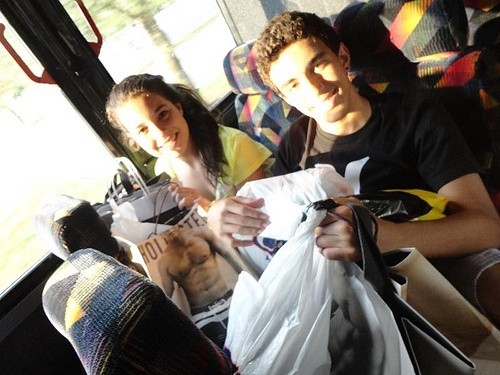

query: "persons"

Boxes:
[104, 74, 272, 211]
[204, 11, 500, 331]
[157, 224, 258, 349]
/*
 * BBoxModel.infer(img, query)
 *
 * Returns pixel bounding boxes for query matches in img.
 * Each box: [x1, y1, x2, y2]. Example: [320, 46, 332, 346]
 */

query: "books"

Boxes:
[111, 203, 256, 349]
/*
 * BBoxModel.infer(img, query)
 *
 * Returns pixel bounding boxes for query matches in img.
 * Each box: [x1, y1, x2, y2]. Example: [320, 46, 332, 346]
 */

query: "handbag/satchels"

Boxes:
[90, 152, 500, 375]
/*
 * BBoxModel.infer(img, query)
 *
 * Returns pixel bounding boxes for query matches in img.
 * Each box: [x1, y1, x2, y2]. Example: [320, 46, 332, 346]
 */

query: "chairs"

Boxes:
[43, 247, 241, 375]
[315, 0, 500, 212]
[219, 40, 304, 159]
[30, 194, 139, 273]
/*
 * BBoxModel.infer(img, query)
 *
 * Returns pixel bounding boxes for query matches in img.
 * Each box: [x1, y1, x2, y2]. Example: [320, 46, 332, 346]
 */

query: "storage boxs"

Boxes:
[96, 161, 478, 375]
